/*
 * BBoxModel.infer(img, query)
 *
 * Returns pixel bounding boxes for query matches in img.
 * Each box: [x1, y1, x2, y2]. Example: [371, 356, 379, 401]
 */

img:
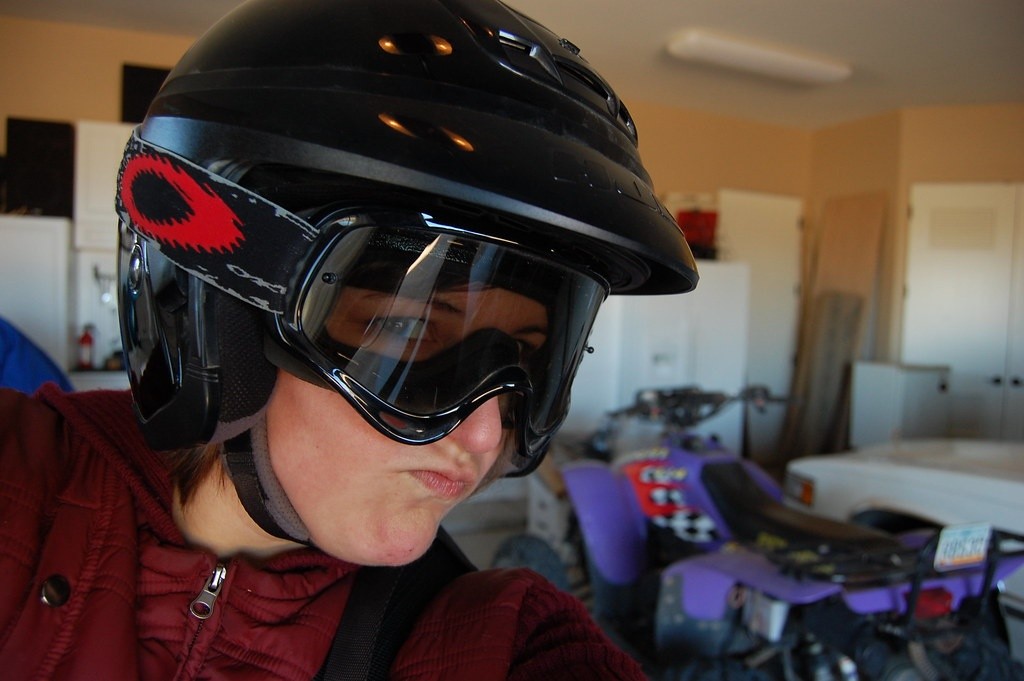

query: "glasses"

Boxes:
[115, 124, 611, 480]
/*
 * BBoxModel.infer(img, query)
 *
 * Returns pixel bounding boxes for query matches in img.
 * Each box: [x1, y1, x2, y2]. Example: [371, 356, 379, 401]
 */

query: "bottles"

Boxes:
[78, 326, 94, 366]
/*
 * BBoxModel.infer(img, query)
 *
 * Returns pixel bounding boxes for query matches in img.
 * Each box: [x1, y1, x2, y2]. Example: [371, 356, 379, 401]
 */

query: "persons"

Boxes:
[0, 0, 699, 681]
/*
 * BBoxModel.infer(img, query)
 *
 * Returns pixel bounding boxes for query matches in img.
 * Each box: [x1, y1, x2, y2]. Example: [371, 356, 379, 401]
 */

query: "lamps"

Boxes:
[665, 26, 858, 90]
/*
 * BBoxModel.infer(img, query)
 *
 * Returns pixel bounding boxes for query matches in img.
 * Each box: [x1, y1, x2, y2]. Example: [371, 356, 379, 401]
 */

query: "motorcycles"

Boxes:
[490, 387, 1024, 681]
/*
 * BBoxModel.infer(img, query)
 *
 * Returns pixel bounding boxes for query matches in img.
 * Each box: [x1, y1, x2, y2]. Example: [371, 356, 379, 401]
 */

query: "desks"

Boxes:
[781, 437, 1024, 540]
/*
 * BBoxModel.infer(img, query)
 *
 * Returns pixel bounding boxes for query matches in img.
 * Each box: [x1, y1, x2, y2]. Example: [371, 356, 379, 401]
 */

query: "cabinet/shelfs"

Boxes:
[525, 467, 573, 563]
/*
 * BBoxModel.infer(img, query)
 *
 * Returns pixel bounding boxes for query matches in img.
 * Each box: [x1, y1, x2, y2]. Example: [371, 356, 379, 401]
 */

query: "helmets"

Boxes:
[110, 0, 699, 452]
